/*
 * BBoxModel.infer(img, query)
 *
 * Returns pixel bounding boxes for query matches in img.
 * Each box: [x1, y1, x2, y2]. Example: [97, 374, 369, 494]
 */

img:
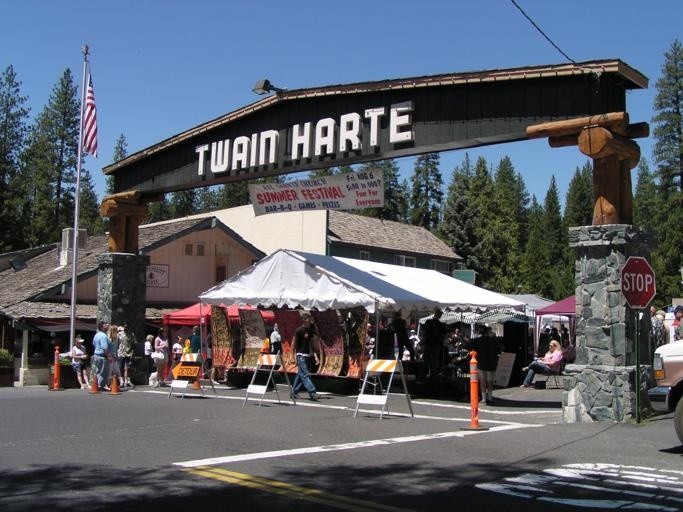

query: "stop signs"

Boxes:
[619, 256, 657, 309]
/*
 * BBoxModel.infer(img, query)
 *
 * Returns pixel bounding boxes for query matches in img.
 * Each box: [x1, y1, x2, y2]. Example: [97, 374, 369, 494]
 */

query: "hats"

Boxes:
[77, 339, 84, 342]
[671, 305, 683, 312]
[655, 310, 666, 317]
[650, 306, 655, 314]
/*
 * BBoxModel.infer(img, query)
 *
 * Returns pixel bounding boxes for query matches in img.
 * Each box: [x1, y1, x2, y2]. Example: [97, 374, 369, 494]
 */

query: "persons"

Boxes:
[71, 320, 137, 392]
[270, 323, 281, 354]
[144, 325, 204, 386]
[639, 306, 683, 352]
[370, 309, 570, 403]
[290, 314, 321, 400]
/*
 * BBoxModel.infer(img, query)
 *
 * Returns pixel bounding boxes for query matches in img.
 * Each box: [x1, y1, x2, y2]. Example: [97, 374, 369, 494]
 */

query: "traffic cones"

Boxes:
[189, 379, 200, 390]
[87, 373, 99, 394]
[107, 374, 123, 395]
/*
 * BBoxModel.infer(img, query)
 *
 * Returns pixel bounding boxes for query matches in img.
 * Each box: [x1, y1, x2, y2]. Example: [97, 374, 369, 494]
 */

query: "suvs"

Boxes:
[646, 338, 683, 447]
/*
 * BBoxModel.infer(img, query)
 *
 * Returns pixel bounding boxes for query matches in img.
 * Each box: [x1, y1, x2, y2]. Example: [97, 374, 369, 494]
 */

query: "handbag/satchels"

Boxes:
[151, 352, 164, 360]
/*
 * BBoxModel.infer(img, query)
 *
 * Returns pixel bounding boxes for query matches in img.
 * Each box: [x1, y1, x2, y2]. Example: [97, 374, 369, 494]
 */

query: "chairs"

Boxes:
[546, 356, 567, 389]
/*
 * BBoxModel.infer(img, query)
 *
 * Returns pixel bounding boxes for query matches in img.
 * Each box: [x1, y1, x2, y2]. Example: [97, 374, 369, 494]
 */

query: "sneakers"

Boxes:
[290, 392, 300, 399]
[520, 385, 528, 388]
[522, 367, 530, 371]
[309, 391, 321, 400]
